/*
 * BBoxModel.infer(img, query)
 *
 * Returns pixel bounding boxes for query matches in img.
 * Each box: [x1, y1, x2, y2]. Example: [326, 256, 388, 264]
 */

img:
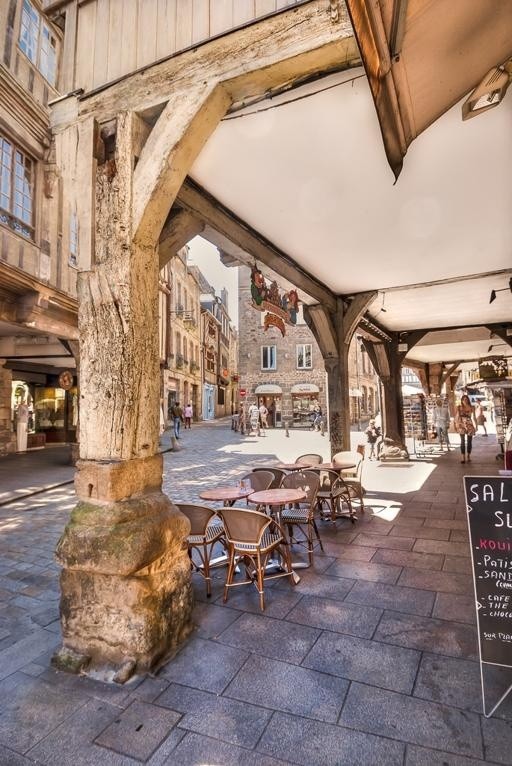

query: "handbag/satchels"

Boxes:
[430, 433, 436, 439]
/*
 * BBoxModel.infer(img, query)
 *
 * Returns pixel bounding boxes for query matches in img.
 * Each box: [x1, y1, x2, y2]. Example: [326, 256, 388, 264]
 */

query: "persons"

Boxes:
[173, 402, 183, 439]
[432, 399, 451, 451]
[313, 402, 322, 432]
[16, 400, 30, 451]
[474, 398, 488, 437]
[183, 404, 193, 429]
[364, 419, 380, 459]
[238, 402, 269, 433]
[454, 395, 478, 463]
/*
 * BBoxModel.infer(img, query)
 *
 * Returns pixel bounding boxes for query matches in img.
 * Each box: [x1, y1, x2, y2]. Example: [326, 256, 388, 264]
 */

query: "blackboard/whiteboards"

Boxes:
[463, 475, 512, 668]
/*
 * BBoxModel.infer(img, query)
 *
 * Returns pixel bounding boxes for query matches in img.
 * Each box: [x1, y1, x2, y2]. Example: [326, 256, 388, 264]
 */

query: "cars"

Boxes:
[467, 394, 493, 410]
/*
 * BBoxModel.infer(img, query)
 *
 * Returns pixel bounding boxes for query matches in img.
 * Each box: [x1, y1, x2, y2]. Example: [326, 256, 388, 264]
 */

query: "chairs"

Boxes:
[175, 451, 366, 611]
